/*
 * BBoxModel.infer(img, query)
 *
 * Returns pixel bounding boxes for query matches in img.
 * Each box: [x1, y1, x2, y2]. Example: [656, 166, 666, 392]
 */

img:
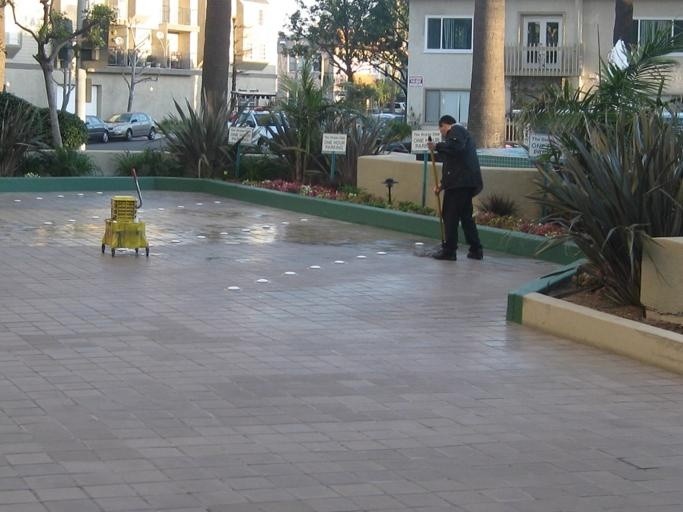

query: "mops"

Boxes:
[425, 136, 446, 258]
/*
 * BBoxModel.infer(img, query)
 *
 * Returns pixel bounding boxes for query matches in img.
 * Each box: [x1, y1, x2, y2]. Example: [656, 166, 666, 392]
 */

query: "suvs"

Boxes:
[226, 110, 298, 155]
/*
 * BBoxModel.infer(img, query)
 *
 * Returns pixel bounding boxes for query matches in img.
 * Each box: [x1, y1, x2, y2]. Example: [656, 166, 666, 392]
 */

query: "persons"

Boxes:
[426, 115, 484, 262]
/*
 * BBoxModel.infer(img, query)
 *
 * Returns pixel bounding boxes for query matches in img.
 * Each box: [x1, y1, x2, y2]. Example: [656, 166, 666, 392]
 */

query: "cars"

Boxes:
[104, 112, 159, 141]
[86, 112, 110, 143]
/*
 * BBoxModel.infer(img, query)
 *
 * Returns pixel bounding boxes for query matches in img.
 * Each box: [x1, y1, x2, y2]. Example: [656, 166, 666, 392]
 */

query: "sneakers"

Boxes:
[433, 246, 457, 260]
[468, 246, 483, 259]
